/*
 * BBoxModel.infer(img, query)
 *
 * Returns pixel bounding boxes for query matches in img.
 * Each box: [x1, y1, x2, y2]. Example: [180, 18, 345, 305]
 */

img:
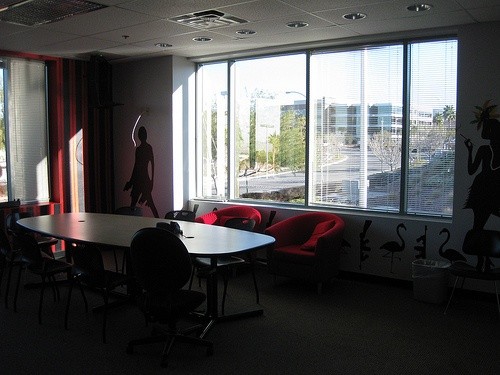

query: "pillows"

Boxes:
[304, 219, 334, 250]
[220, 216, 252, 225]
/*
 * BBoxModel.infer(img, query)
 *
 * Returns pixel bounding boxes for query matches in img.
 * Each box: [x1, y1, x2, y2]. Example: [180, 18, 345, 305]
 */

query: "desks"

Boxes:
[16, 213, 277, 341]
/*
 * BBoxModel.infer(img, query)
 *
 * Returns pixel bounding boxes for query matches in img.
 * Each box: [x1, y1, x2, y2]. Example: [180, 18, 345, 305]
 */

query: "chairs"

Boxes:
[0, 206, 347, 365]
[443, 228, 500, 316]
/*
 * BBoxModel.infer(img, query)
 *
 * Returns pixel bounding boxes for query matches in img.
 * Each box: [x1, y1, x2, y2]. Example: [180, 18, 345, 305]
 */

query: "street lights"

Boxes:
[260, 123, 273, 180]
[372, 115, 383, 172]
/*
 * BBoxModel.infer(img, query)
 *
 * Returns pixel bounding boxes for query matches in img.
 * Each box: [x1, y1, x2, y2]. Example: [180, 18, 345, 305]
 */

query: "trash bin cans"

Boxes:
[412, 258, 451, 302]
